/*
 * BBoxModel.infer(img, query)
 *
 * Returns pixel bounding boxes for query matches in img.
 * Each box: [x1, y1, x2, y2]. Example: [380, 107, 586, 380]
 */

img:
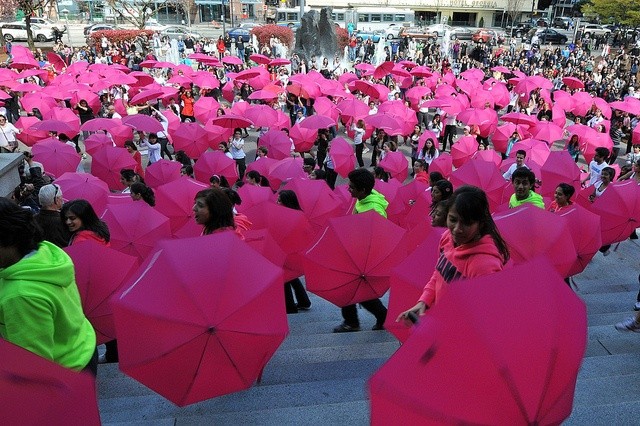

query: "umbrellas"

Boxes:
[558, 204, 601, 277]
[111, 232, 291, 407]
[489, 203, 578, 282]
[52, 172, 110, 215]
[368, 254, 588, 426]
[153, 175, 209, 237]
[382, 223, 449, 344]
[590, 180, 640, 248]
[99, 198, 173, 263]
[0, 336, 102, 426]
[62, 239, 141, 347]
[387, 182, 432, 247]
[301, 210, 417, 308]
[193, 187, 252, 240]
[238, 202, 317, 284]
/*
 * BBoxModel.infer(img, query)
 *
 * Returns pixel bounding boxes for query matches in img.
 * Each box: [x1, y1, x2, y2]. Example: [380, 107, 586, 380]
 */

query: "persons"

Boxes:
[508, 167, 546, 210]
[615, 274, 639, 334]
[61, 199, 118, 363]
[120, 166, 145, 193]
[429, 180, 453, 207]
[128, 186, 156, 207]
[546, 182, 575, 286]
[47, 28, 256, 69]
[38, 184, 74, 248]
[333, 168, 388, 332]
[277, 190, 312, 313]
[395, 185, 510, 324]
[351, 14, 637, 101]
[431, 199, 455, 228]
[589, 167, 615, 256]
[0, 196, 99, 380]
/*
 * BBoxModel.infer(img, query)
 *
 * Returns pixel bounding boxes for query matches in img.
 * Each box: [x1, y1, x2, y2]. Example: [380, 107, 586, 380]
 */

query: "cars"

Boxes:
[613, 27, 640, 41]
[225, 26, 251, 43]
[383, 25, 402, 39]
[156, 26, 202, 42]
[236, 22, 261, 32]
[602, 24, 618, 31]
[400, 26, 438, 44]
[579, 23, 611, 34]
[531, 28, 568, 45]
[427, 23, 454, 36]
[16, 17, 66, 34]
[1, 21, 54, 42]
[84, 22, 127, 36]
[507, 22, 535, 37]
[572, 16, 588, 28]
[473, 29, 505, 44]
[537, 17, 550, 27]
[451, 27, 475, 40]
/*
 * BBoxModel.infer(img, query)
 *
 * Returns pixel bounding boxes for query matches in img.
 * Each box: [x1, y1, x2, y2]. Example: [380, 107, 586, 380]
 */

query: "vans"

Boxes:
[553, 16, 571, 28]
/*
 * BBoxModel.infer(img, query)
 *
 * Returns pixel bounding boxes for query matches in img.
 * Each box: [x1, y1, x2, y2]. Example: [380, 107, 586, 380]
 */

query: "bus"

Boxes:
[272, 5, 415, 39]
